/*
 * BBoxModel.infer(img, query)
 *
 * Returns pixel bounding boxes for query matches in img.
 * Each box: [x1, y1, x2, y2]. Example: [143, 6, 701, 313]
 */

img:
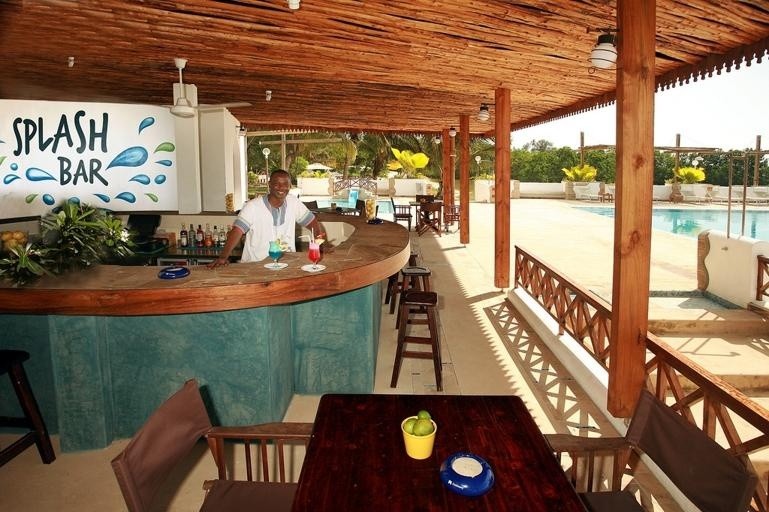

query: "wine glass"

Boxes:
[308, 241, 322, 270]
[268, 241, 282, 268]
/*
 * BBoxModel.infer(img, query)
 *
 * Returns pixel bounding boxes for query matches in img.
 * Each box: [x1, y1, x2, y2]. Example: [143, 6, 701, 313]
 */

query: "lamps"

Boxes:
[433, 133, 442, 145]
[447, 123, 458, 138]
[478, 102, 495, 122]
[584, 25, 620, 78]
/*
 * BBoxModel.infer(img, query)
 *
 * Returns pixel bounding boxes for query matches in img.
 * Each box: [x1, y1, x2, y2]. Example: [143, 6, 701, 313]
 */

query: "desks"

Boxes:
[316, 206, 360, 216]
[289, 391, 590, 511]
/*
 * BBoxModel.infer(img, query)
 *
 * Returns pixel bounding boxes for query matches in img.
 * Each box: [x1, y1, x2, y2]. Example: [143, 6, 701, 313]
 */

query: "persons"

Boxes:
[205, 168, 328, 270]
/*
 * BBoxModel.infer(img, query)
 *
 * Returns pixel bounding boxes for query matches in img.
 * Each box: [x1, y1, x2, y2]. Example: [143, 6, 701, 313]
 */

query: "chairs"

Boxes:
[302, 201, 318, 213]
[110, 375, 319, 511]
[357, 199, 366, 217]
[670, 182, 769, 208]
[574, 186, 603, 203]
[391, 195, 461, 237]
[539, 390, 758, 509]
[598, 183, 613, 202]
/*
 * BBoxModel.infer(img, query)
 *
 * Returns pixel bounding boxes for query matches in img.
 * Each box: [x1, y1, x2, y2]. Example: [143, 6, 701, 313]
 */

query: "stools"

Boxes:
[2, 349, 55, 471]
[389, 288, 444, 391]
[394, 265, 435, 329]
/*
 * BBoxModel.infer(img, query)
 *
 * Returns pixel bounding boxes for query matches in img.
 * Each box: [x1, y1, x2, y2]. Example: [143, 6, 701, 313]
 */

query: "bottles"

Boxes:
[180, 222, 232, 246]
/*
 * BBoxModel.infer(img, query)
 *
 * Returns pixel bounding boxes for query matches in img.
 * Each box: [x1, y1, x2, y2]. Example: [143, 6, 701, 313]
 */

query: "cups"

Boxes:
[401, 414, 437, 459]
[330, 203, 337, 209]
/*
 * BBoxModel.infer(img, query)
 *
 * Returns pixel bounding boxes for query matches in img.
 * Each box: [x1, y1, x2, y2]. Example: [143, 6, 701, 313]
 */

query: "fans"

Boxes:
[134, 55, 252, 120]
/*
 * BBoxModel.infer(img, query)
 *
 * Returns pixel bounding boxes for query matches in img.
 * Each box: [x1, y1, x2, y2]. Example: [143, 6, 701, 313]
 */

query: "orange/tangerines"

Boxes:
[404, 411, 434, 436]
